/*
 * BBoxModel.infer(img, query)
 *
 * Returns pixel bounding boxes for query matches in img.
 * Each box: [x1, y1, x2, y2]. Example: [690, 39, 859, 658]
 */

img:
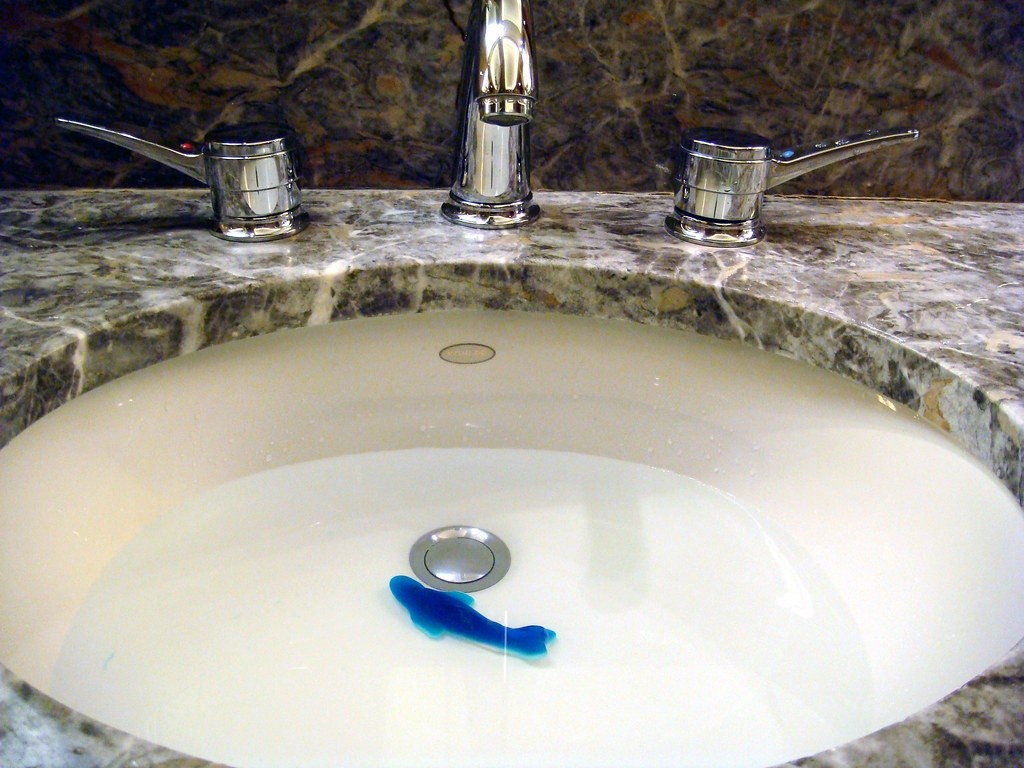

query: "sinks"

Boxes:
[0, 254, 1023, 768]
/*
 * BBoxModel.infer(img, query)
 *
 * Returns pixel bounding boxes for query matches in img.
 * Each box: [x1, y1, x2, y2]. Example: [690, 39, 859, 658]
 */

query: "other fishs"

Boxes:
[388, 575, 555, 660]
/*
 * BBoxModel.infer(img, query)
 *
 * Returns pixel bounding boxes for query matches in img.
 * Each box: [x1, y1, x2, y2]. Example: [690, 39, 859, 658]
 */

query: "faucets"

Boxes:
[441, 0, 538, 227]
